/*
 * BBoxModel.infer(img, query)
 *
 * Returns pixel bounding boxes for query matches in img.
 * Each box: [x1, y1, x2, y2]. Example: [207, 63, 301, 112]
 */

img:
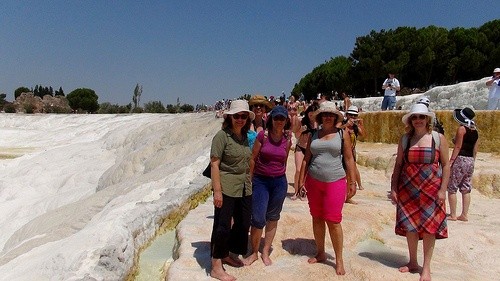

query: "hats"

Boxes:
[402, 104, 435, 125]
[453, 107, 475, 127]
[312, 101, 344, 122]
[344, 106, 360, 118]
[272, 106, 288, 118]
[417, 98, 430, 108]
[223, 100, 255, 121]
[494, 68, 500, 72]
[247, 95, 272, 109]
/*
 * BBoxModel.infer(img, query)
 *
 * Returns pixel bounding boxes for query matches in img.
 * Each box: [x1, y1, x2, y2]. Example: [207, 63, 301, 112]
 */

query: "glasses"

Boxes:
[231, 113, 247, 120]
[349, 114, 357, 117]
[410, 115, 425, 120]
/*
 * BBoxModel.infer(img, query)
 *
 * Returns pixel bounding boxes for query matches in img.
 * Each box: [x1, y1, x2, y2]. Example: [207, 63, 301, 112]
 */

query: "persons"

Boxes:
[391, 104, 450, 281]
[486, 68, 500, 110]
[206, 98, 232, 118]
[381, 71, 400, 111]
[248, 96, 273, 133]
[264, 90, 365, 205]
[298, 101, 356, 275]
[242, 106, 292, 265]
[449, 107, 478, 221]
[416, 97, 444, 135]
[210, 100, 252, 281]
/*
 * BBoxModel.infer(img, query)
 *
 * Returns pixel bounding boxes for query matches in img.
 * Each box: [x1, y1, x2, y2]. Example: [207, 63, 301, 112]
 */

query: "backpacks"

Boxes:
[433, 118, 444, 135]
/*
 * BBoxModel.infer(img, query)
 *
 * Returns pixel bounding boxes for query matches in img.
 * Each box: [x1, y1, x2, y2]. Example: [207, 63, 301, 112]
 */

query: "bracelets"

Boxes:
[296, 184, 304, 196]
[350, 180, 357, 187]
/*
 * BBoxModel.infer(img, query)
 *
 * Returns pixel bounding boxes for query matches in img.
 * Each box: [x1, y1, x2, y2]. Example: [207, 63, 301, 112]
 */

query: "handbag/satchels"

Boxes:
[203, 162, 211, 178]
